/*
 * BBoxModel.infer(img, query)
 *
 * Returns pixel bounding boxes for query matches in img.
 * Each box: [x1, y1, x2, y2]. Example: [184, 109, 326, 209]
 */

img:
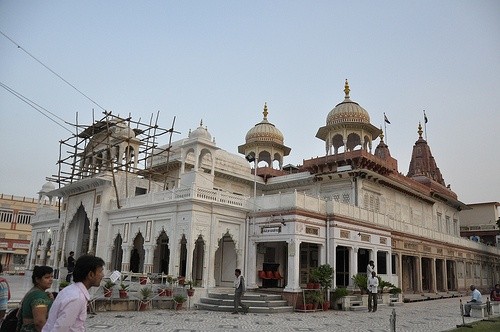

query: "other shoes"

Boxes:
[368, 309, 372, 312]
[231, 311, 238, 314]
[244, 306, 250, 313]
[463, 315, 471, 317]
[372, 309, 377, 312]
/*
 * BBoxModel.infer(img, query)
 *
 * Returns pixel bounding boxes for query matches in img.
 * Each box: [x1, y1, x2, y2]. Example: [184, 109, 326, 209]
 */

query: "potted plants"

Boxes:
[103, 280, 116, 297]
[119, 277, 129, 299]
[352, 274, 368, 294]
[158, 274, 195, 310]
[139, 271, 147, 284]
[301, 264, 350, 311]
[139, 286, 153, 310]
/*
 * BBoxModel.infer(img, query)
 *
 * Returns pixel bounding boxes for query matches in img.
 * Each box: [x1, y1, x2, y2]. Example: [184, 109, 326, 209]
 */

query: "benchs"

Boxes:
[471, 301, 500, 318]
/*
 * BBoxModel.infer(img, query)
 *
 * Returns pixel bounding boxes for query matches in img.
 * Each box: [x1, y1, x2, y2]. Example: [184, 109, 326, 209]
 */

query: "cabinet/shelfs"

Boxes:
[295, 288, 323, 313]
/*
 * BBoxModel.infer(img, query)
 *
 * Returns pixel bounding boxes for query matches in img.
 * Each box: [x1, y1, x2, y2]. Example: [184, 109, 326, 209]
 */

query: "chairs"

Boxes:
[258, 270, 284, 288]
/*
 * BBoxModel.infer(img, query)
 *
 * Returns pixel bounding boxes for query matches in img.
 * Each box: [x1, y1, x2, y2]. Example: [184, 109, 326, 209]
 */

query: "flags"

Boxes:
[384, 114, 391, 124]
[424, 111, 428, 123]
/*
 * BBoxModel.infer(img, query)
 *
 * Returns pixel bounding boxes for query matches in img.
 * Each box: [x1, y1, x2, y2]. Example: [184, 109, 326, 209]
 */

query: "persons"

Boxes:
[0, 263, 11, 323]
[480, 238, 497, 246]
[471, 234, 478, 241]
[367, 271, 379, 312]
[490, 284, 500, 301]
[66, 251, 75, 283]
[16, 265, 54, 332]
[366, 260, 375, 294]
[463, 284, 482, 317]
[40, 255, 106, 332]
[231, 269, 250, 314]
